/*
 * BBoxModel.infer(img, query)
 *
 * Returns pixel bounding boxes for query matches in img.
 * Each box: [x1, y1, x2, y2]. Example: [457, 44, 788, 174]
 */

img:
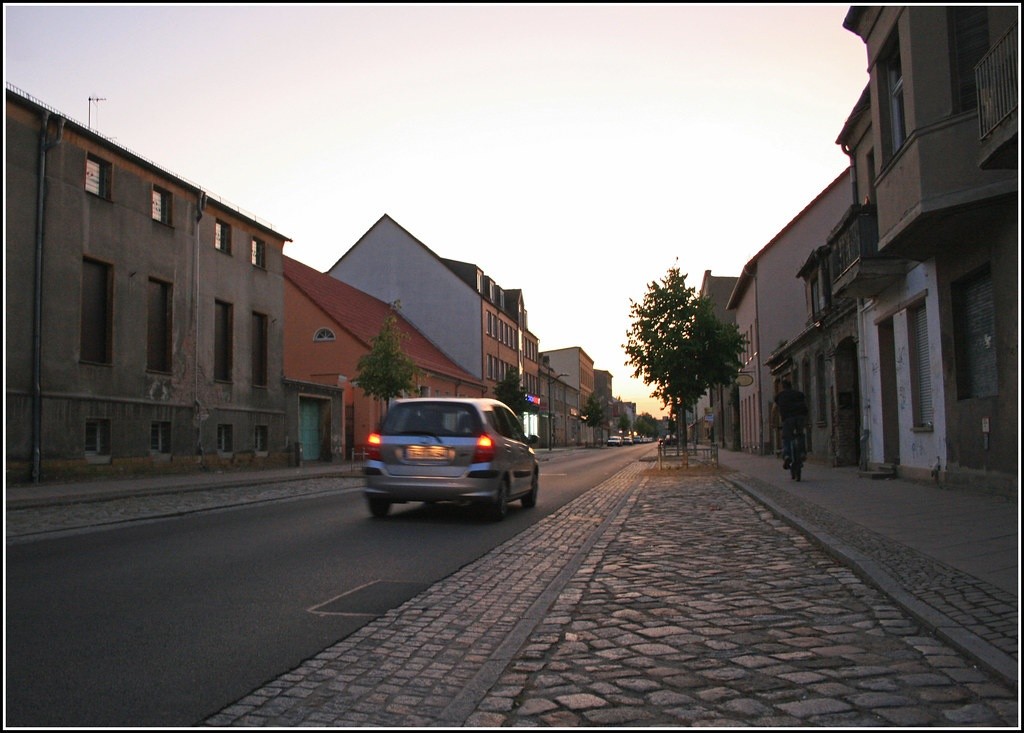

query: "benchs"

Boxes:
[657, 437, 718, 471]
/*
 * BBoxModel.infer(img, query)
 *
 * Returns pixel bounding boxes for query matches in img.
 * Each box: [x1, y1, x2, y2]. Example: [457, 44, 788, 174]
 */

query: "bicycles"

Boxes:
[774, 422, 810, 482]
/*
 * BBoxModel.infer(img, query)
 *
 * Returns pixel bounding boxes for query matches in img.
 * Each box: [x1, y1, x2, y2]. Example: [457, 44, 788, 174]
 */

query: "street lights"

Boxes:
[548, 373, 570, 451]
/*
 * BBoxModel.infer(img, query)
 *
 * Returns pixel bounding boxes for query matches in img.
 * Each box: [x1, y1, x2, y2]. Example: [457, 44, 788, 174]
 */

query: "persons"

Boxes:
[771, 379, 809, 470]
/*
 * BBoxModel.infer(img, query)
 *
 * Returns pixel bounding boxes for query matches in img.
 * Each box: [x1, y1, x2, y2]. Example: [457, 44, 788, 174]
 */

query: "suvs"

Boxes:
[607, 436, 624, 447]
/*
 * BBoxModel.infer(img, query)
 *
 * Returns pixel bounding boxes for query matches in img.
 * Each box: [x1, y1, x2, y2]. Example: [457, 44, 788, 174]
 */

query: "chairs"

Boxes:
[455, 413, 475, 433]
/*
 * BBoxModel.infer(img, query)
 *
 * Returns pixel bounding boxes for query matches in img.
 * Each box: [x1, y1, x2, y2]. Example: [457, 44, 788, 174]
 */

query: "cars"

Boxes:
[623, 436, 633, 445]
[361, 397, 540, 521]
[664, 435, 678, 445]
[633, 435, 642, 443]
[642, 436, 652, 443]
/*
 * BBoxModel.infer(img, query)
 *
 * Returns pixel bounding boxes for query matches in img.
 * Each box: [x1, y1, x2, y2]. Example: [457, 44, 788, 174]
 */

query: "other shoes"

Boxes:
[800, 452, 807, 461]
[783, 455, 791, 470]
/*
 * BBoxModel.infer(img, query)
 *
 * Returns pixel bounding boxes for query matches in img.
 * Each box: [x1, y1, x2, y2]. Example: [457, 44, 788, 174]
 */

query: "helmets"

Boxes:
[782, 378, 792, 388]
